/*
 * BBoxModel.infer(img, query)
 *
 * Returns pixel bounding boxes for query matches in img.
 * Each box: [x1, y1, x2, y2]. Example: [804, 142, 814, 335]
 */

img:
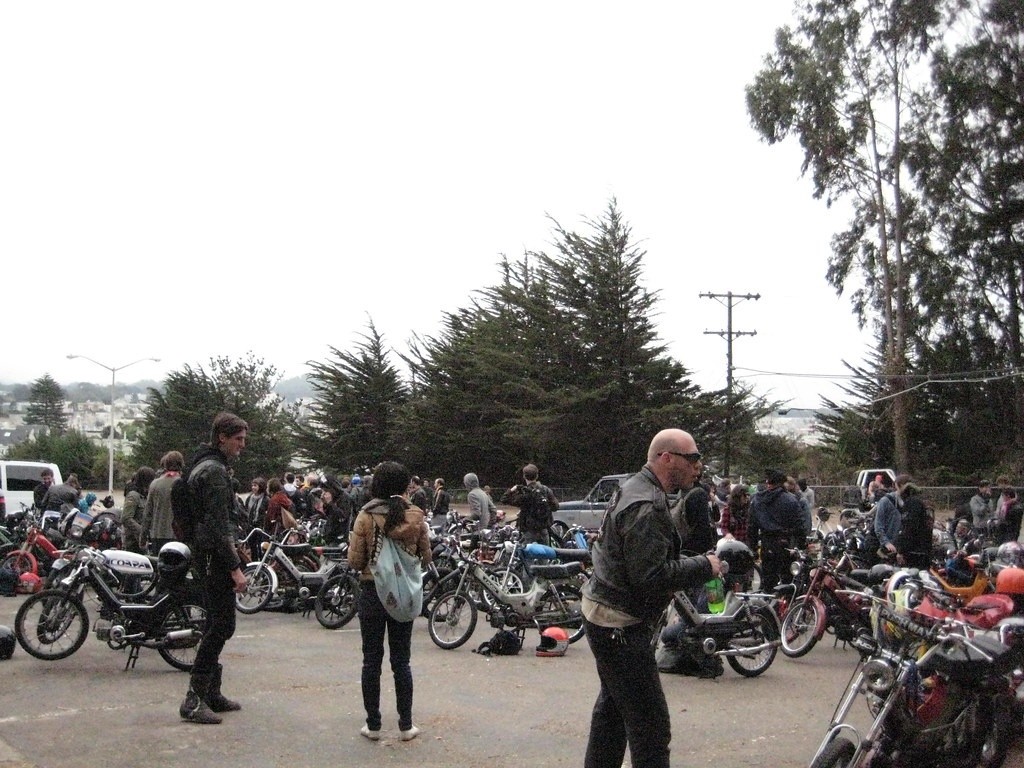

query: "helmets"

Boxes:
[0, 624, 16, 659]
[158, 542, 191, 582]
[996, 567, 1024, 594]
[868, 564, 893, 585]
[17, 573, 39, 593]
[536, 627, 568, 657]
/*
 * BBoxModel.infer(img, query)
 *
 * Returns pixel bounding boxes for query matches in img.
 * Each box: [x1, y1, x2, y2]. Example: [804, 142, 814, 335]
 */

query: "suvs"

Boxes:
[552, 474, 687, 540]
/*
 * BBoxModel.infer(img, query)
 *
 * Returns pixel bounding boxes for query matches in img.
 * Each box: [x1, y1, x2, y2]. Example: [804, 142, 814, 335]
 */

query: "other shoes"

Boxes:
[360, 724, 379, 739]
[401, 724, 419, 740]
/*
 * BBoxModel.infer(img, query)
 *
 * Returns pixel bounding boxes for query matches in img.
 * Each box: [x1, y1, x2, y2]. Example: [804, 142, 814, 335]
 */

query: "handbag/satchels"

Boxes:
[374, 536, 423, 622]
[281, 506, 297, 529]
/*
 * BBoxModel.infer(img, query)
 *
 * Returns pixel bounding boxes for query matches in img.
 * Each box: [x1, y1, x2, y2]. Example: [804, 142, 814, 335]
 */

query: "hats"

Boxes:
[321, 480, 342, 494]
[765, 469, 787, 484]
[100, 496, 113, 503]
[352, 474, 360, 483]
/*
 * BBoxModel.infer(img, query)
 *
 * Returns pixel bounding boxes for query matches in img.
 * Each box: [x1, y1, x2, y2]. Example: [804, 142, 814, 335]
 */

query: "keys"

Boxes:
[206, 566, 211, 576]
[610, 627, 627, 645]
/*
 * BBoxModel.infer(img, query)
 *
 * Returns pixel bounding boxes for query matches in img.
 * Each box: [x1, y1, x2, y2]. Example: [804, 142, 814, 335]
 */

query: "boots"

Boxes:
[204, 664, 240, 711]
[180, 672, 222, 724]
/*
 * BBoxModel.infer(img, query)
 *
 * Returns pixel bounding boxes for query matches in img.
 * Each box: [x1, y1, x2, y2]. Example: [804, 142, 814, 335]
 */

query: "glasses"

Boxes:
[657, 452, 701, 463]
[744, 494, 750, 497]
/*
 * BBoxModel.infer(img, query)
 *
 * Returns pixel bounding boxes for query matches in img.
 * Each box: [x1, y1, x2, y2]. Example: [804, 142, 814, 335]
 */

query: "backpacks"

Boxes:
[520, 485, 552, 530]
[476, 630, 521, 655]
[170, 457, 226, 543]
[670, 487, 705, 544]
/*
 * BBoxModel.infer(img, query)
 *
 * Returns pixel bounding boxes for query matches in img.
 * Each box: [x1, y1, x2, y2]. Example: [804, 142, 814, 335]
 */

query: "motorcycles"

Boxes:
[810, 566, 1024, 768]
[14, 508, 210, 671]
[0, 500, 127, 592]
[235, 519, 363, 629]
[420, 508, 597, 649]
[654, 502, 1024, 678]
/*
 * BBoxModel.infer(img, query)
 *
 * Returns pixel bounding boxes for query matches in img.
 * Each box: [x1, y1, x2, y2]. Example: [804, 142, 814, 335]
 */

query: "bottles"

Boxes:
[704, 577, 725, 613]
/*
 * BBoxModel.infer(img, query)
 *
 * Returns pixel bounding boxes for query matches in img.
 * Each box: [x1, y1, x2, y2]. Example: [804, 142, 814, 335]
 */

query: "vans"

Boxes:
[852, 470, 898, 500]
[0, 460, 64, 515]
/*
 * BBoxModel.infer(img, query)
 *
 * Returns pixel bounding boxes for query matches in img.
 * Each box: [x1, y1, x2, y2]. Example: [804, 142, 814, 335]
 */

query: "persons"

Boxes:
[579, 427, 720, 768]
[228, 466, 450, 562]
[34, 469, 114, 578]
[867, 475, 1024, 570]
[670, 469, 814, 600]
[464, 473, 497, 555]
[348, 461, 432, 741]
[180, 412, 248, 724]
[500, 463, 559, 588]
[119, 451, 185, 605]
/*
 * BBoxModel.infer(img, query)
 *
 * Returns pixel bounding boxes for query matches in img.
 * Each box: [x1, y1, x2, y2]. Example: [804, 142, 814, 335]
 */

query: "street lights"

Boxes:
[66, 352, 160, 499]
